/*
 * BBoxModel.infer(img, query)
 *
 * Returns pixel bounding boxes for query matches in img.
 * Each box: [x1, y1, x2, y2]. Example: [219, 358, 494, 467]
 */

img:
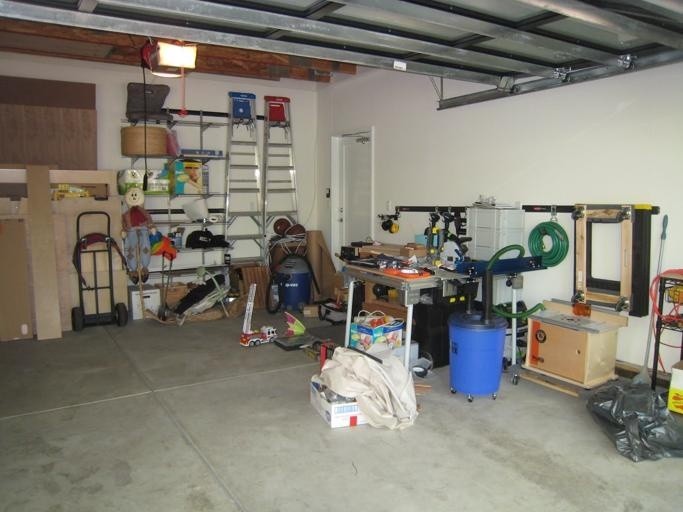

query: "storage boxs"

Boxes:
[155, 282, 188, 306]
[521, 307, 624, 389]
[128, 284, 160, 320]
[667, 360, 683, 415]
[121, 127, 168, 156]
[309, 381, 368, 429]
[392, 338, 419, 367]
[465, 207, 525, 305]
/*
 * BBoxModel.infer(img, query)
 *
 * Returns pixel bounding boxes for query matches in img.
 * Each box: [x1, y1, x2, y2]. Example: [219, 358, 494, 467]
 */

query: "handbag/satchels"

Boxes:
[350, 310, 404, 351]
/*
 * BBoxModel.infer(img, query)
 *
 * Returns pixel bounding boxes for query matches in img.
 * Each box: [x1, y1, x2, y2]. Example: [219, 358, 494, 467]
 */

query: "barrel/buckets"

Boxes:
[447, 310, 509, 396]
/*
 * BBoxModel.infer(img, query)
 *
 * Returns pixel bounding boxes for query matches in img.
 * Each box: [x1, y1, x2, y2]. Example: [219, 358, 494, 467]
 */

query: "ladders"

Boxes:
[221, 91, 299, 289]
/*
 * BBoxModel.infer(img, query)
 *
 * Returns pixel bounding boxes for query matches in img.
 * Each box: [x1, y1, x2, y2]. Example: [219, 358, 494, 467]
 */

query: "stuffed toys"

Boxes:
[148, 227, 177, 260]
[48, 183, 89, 203]
[119, 187, 157, 284]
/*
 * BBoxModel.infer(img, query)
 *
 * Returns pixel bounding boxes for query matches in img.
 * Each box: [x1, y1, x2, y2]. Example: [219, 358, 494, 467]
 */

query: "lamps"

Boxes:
[155, 42, 197, 70]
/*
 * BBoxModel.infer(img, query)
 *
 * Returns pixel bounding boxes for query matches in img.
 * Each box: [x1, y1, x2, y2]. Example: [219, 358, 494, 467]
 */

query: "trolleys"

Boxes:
[69, 208, 128, 330]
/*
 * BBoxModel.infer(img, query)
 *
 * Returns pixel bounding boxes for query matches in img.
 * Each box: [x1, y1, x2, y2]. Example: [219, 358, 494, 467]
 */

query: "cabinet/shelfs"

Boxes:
[120, 118, 230, 273]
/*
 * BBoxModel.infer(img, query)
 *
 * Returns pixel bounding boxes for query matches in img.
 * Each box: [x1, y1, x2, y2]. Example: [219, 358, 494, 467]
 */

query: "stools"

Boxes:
[651, 272, 683, 392]
